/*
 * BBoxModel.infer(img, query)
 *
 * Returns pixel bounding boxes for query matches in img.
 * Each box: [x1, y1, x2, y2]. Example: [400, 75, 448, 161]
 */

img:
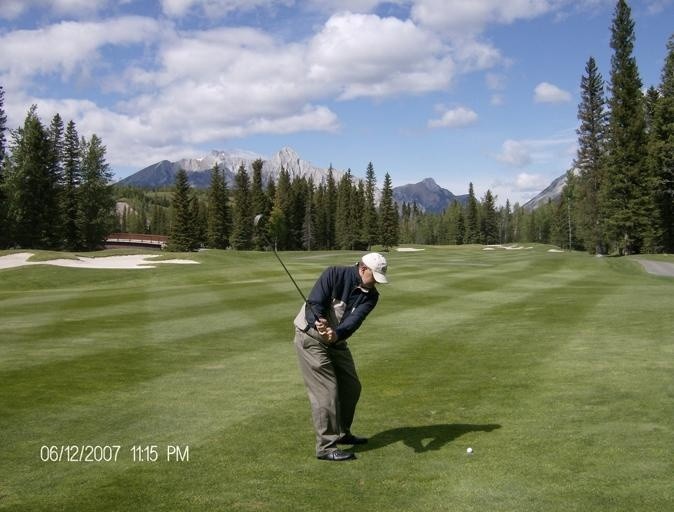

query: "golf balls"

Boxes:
[466, 448, 471, 451]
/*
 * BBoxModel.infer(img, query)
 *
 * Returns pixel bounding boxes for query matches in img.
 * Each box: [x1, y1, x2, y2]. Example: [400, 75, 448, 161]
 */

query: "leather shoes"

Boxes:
[317, 451, 354, 461]
[337, 436, 369, 445]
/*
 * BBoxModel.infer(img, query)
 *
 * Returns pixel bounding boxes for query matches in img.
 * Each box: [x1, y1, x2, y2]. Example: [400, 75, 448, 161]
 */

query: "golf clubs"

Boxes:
[254, 215, 321, 323]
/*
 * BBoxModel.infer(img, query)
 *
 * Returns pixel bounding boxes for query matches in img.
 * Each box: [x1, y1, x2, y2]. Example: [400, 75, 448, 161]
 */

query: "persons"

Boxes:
[291, 251, 389, 460]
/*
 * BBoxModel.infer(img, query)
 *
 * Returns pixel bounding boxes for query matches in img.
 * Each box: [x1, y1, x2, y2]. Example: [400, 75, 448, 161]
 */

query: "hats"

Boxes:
[362, 252, 388, 284]
